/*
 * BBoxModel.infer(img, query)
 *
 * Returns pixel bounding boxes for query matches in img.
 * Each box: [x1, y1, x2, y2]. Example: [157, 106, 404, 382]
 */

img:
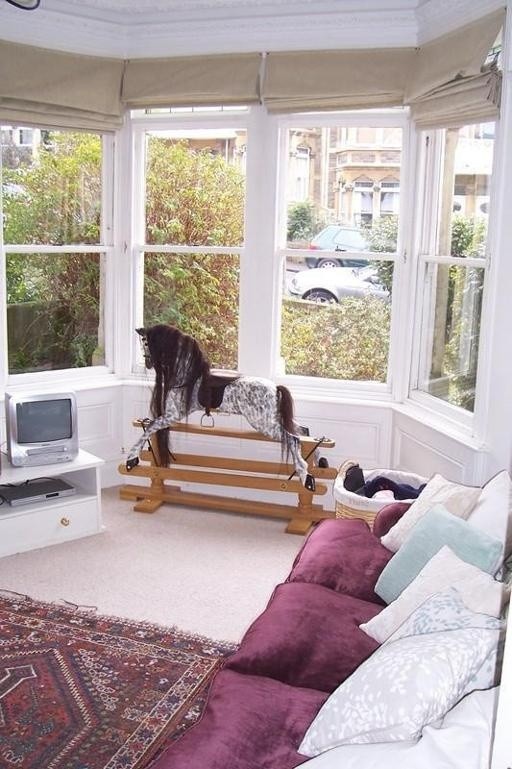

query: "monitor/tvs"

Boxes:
[5, 388, 79, 467]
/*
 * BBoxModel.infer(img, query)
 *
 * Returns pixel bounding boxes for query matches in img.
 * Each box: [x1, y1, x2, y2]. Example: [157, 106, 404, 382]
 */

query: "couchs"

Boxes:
[153, 471, 511, 768]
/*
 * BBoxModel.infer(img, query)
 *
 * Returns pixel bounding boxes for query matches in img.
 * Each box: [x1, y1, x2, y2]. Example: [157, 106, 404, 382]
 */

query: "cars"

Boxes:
[289, 264, 391, 303]
[306, 226, 370, 269]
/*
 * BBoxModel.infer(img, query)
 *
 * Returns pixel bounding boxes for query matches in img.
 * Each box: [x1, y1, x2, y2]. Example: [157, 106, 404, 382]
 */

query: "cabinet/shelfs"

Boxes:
[0, 448, 105, 558]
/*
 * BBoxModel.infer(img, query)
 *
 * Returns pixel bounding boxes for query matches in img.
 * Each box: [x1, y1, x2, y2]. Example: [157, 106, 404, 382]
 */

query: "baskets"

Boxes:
[334, 459, 428, 532]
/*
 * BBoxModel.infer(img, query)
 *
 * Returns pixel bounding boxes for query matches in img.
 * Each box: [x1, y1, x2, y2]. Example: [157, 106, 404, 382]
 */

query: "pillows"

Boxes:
[359, 544, 509, 645]
[381, 473, 483, 554]
[380, 586, 506, 702]
[373, 503, 506, 605]
[298, 626, 506, 757]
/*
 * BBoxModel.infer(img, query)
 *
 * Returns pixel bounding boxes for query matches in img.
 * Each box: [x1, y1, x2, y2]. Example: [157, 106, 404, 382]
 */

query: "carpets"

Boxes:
[0, 591, 238, 766]
